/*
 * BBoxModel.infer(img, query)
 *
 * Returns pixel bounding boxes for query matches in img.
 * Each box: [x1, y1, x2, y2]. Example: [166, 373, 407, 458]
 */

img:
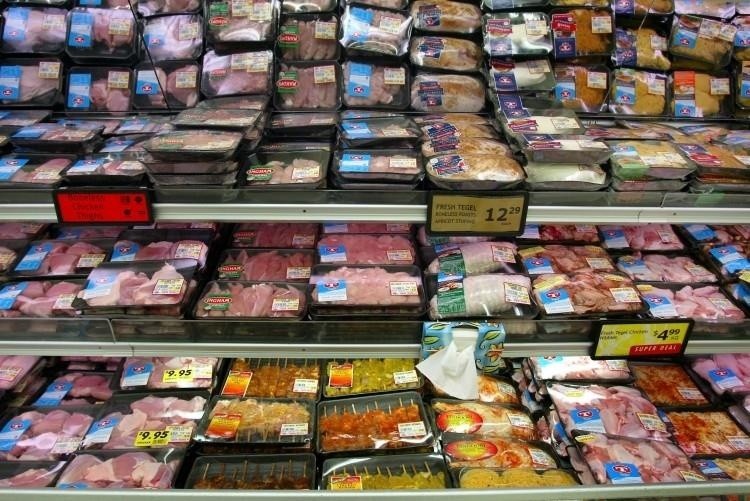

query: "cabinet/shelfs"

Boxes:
[0, 193, 750, 501]
